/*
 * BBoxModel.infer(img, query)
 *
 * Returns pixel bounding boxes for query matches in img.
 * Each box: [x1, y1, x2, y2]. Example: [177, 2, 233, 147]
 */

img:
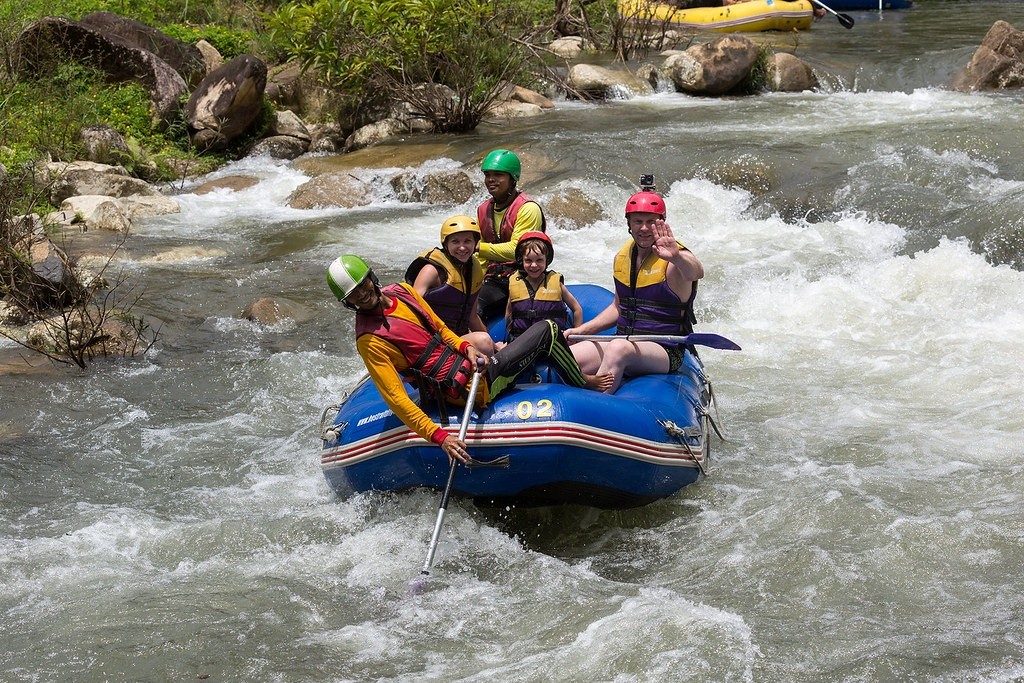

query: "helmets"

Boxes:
[441, 215, 482, 244]
[625, 191, 666, 222]
[481, 148, 521, 181]
[515, 231, 554, 266]
[325, 254, 372, 303]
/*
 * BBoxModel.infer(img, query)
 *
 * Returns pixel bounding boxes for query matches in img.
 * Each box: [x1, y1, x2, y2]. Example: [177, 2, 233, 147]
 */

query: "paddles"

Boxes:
[419, 357, 486, 577]
[810, 0, 855, 29]
[567, 332, 742, 352]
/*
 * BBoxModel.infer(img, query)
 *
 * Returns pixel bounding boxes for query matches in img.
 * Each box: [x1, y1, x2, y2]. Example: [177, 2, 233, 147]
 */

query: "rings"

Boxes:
[456, 447, 461, 452]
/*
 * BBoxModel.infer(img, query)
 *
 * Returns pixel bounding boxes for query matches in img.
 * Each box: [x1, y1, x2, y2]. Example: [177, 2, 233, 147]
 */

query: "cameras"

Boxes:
[639, 174, 655, 186]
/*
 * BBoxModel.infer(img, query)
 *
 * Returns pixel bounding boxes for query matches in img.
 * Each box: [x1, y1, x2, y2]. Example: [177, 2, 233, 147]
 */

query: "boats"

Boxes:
[318, 285, 712, 511]
[616, 0, 813, 32]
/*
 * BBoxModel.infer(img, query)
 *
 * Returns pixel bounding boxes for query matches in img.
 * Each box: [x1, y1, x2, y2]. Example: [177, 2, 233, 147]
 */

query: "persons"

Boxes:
[477, 150, 546, 326]
[326, 256, 615, 468]
[405, 215, 508, 357]
[562, 191, 704, 396]
[505, 229, 582, 344]
